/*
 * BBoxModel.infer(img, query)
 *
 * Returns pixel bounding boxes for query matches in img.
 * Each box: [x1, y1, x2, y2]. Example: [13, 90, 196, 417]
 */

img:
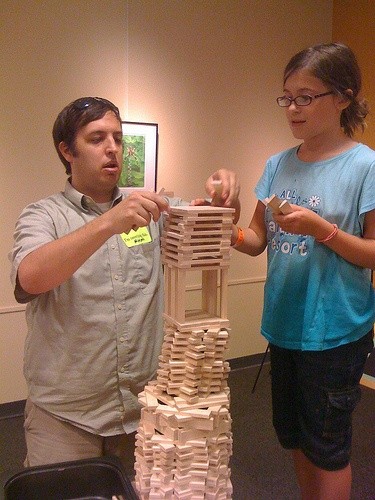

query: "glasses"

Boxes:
[276, 91, 332, 107]
[64, 97, 98, 140]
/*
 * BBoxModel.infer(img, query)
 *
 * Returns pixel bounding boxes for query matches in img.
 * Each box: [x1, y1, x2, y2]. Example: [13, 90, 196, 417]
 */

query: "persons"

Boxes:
[8, 97, 241, 470]
[186, 42, 375, 500]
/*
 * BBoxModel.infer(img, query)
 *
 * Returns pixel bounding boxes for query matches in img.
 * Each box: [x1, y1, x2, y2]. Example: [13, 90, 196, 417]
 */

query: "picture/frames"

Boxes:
[116, 121, 157, 199]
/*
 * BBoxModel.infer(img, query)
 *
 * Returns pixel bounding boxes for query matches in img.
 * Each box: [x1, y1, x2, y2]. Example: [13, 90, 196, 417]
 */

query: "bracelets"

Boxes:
[314, 222, 338, 243]
[230, 226, 245, 249]
